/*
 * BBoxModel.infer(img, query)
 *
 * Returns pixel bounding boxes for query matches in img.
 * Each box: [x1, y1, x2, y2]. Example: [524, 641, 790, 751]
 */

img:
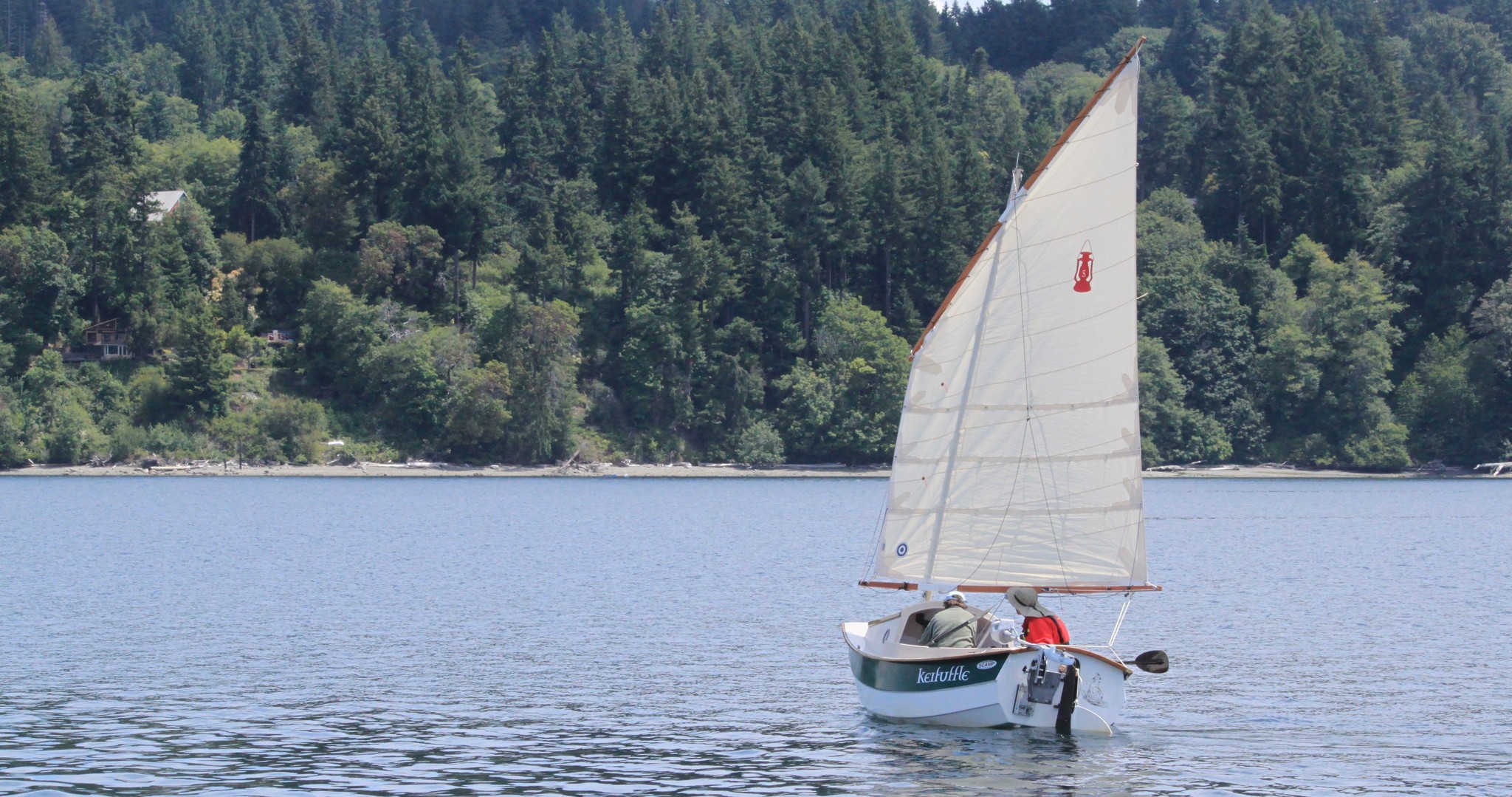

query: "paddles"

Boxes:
[1115, 649, 1169, 674]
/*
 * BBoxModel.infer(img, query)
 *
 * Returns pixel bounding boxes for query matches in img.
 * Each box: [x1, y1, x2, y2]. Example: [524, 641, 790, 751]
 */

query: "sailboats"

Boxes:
[818, 31, 1164, 748]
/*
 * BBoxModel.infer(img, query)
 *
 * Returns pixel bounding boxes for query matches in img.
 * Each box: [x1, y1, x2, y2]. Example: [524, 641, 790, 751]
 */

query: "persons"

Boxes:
[918, 591, 977, 648]
[1007, 587, 1070, 645]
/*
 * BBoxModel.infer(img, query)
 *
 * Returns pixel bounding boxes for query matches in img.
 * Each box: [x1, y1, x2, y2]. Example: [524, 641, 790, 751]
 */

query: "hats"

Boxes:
[1006, 587, 1056, 618]
[945, 591, 968, 604]
